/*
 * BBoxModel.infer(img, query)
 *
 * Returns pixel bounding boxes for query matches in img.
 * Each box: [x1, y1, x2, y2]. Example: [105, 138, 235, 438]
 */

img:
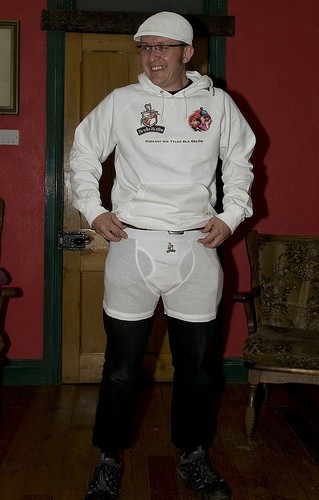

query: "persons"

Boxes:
[69, 12, 256, 500]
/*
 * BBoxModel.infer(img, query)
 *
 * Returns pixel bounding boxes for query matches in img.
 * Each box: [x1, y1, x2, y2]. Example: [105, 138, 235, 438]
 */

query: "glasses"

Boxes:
[136, 44, 190, 52]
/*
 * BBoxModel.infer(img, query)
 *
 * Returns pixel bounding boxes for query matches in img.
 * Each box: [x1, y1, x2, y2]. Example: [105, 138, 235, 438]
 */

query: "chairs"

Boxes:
[233, 228, 319, 440]
[0, 196, 23, 389]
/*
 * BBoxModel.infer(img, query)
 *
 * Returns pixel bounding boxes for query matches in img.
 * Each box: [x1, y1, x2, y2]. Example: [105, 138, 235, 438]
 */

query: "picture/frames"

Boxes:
[0, 19, 20, 116]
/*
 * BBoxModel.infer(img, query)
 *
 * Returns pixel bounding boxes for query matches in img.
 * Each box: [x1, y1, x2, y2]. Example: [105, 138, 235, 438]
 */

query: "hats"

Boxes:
[134, 11, 193, 47]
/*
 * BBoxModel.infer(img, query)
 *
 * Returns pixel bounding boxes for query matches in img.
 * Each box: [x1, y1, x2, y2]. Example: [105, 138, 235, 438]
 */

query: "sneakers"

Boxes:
[176, 446, 231, 500]
[84, 453, 123, 500]
